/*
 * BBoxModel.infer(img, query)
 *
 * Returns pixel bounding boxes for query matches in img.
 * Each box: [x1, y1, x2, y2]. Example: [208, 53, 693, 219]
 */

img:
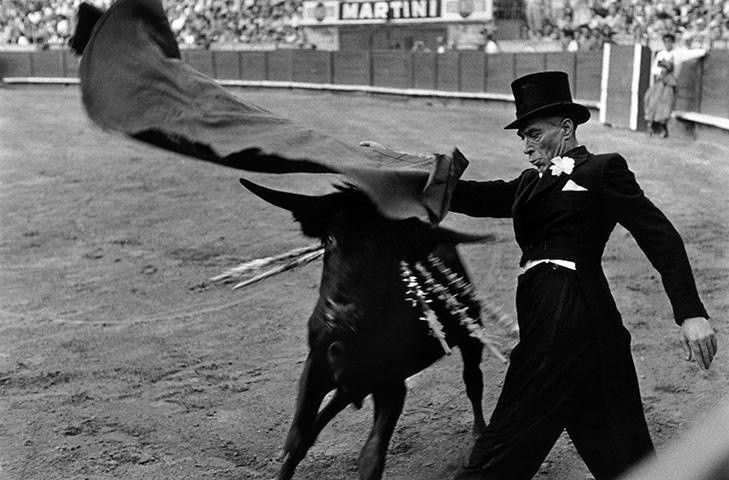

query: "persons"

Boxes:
[358, 70, 717, 479]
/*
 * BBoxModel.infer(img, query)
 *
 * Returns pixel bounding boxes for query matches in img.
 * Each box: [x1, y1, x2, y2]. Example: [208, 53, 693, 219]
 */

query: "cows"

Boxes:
[240, 177, 494, 479]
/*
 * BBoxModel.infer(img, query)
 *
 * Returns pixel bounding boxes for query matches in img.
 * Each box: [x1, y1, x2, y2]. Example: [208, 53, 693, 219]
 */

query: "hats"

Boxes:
[504, 70, 592, 135]
